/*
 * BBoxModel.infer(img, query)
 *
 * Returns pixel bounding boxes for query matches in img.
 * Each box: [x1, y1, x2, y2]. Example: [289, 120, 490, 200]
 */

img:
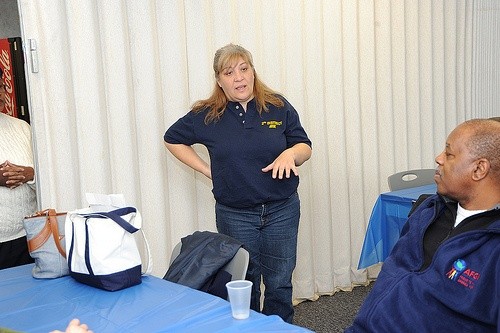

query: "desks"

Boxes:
[0, 263, 316, 333]
[381, 182, 437, 261]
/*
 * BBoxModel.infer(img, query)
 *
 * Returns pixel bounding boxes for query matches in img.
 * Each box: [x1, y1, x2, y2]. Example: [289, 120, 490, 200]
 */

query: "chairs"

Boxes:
[170, 230, 250, 302]
[387, 169, 436, 190]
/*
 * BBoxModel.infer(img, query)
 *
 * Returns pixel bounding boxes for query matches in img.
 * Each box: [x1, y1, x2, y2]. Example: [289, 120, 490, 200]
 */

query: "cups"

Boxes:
[226, 280, 253, 320]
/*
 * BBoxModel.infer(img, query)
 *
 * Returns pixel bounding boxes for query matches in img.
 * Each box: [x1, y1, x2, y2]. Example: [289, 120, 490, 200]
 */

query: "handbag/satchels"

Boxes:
[22, 208, 70, 279]
[64, 205, 152, 292]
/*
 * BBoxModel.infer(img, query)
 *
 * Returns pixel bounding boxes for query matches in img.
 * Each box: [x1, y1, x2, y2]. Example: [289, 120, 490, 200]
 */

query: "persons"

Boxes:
[0, 68, 37, 270]
[164, 43, 312, 323]
[344, 119, 500, 333]
